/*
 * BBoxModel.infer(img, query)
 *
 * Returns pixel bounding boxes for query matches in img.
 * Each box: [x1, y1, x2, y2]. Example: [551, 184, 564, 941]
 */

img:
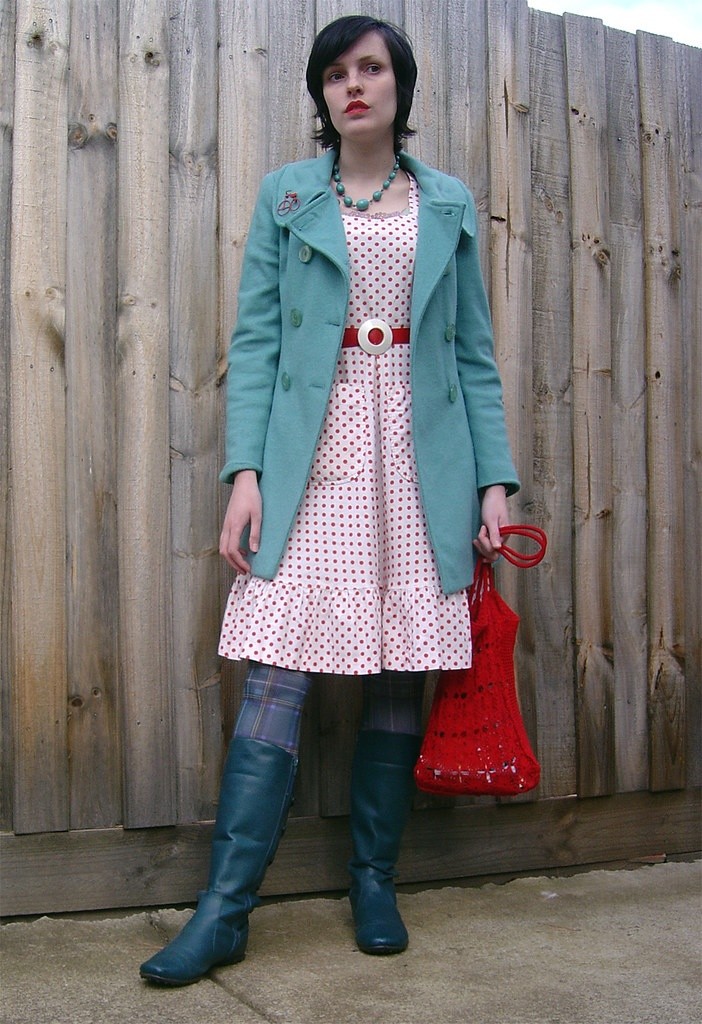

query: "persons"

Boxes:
[140, 16, 522, 988]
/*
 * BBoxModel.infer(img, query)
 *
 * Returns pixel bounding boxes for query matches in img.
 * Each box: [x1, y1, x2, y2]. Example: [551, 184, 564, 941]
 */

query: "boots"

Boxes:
[349, 727, 423, 955]
[139, 736, 299, 985]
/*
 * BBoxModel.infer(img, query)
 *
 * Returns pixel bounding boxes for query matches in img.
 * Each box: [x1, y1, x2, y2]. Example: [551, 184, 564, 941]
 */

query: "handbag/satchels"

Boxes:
[413, 524, 547, 796]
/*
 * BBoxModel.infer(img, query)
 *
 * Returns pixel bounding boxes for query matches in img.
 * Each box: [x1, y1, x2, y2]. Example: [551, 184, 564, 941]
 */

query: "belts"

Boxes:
[341, 319, 410, 355]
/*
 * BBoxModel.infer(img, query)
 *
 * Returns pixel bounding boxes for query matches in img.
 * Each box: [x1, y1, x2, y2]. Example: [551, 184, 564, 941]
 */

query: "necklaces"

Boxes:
[334, 154, 401, 212]
